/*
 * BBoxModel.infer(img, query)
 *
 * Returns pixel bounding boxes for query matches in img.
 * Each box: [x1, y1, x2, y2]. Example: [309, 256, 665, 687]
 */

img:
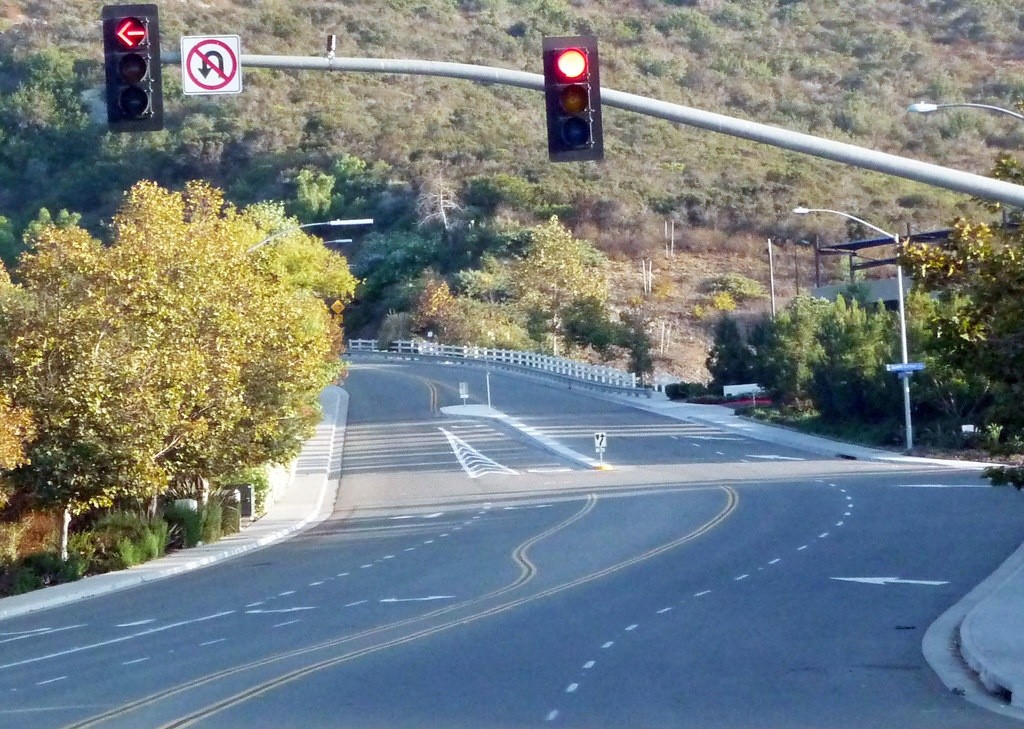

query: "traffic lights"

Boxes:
[541, 36, 604, 164]
[102, 4, 164, 134]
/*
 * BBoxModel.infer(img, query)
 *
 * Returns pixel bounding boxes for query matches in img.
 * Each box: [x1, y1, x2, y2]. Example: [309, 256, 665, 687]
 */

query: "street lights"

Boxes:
[792, 206, 913, 450]
